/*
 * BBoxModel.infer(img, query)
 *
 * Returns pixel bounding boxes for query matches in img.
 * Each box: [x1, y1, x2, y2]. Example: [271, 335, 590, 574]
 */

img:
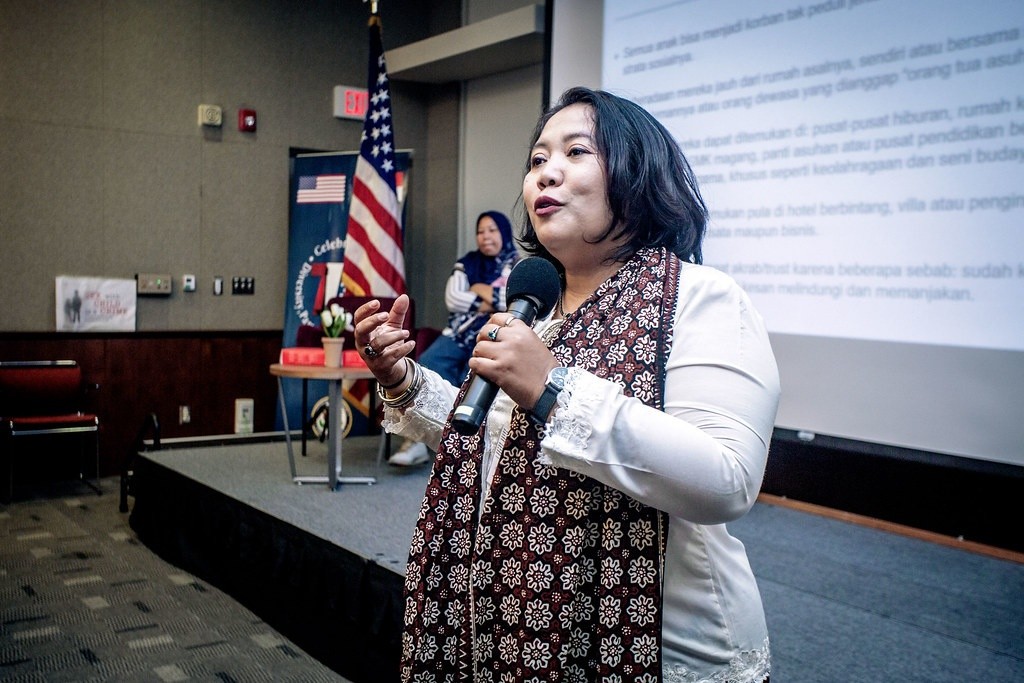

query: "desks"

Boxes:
[269, 363, 390, 492]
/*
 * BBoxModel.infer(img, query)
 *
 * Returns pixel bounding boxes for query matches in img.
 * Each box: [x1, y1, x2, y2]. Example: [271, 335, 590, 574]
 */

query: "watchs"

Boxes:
[531, 367, 568, 424]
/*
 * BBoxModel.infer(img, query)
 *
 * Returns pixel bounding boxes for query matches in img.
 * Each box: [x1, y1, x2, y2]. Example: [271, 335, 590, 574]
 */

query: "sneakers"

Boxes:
[388, 441, 431, 465]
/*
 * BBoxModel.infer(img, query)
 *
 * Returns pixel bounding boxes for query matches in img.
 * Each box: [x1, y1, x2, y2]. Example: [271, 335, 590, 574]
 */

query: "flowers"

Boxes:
[320, 304, 355, 338]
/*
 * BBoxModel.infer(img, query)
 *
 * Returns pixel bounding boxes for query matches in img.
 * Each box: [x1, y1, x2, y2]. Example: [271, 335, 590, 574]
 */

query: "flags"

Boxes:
[341, 16, 406, 418]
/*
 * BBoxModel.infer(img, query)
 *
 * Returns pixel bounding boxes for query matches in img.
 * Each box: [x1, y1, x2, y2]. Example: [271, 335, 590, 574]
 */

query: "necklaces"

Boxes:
[559, 296, 570, 317]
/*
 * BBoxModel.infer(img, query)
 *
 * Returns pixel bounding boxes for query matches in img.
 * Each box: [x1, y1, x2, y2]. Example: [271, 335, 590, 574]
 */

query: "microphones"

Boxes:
[452, 256, 561, 437]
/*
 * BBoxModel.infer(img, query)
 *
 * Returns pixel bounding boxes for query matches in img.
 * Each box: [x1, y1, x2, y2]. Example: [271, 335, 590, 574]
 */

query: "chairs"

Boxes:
[0, 359, 104, 505]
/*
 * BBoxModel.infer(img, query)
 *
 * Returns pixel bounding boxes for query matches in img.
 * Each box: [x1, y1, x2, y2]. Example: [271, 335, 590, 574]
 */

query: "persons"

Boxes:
[388, 211, 520, 467]
[63, 290, 81, 323]
[353, 87, 782, 683]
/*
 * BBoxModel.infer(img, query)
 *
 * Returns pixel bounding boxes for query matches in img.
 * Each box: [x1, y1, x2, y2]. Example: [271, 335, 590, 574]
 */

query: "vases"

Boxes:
[321, 337, 345, 369]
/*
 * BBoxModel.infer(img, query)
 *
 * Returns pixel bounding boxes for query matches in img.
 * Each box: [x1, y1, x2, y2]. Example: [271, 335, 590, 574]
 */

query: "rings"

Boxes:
[505, 317, 514, 327]
[488, 326, 502, 341]
[364, 342, 386, 358]
